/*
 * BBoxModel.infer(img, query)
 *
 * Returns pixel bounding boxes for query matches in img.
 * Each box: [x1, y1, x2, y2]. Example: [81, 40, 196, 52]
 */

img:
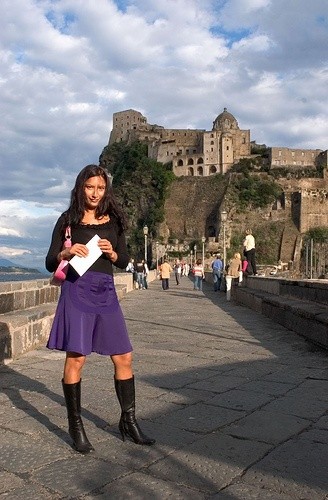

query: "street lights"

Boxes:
[143, 224, 149, 263]
[220, 210, 227, 291]
[201, 234, 206, 271]
[190, 250, 193, 268]
[156, 241, 159, 270]
[193, 245, 197, 264]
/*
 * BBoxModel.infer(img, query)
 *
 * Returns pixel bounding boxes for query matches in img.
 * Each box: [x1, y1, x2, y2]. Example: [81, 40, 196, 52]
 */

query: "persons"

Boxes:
[243, 229, 258, 276]
[225, 252, 242, 302]
[126, 255, 254, 292]
[45, 164, 156, 453]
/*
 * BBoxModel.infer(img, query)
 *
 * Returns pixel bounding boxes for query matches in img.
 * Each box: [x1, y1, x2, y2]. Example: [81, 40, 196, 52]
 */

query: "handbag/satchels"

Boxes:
[50, 225, 72, 286]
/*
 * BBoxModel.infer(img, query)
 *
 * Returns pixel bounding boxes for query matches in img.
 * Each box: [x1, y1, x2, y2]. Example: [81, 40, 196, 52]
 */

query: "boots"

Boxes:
[113, 374, 156, 445]
[61, 378, 95, 454]
